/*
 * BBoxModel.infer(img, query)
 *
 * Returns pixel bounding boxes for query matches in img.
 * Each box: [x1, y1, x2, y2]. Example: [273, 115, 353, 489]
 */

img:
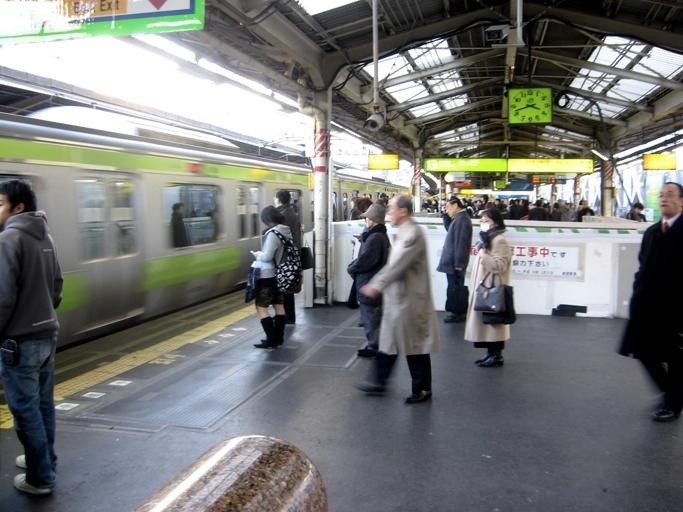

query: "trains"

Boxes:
[0, 111, 411, 368]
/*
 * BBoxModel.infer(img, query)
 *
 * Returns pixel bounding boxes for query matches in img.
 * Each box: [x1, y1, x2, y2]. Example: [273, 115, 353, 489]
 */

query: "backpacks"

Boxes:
[267, 229, 302, 294]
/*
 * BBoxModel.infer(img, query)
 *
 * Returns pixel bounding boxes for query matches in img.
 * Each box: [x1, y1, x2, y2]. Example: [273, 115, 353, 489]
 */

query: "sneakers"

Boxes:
[14, 454, 51, 494]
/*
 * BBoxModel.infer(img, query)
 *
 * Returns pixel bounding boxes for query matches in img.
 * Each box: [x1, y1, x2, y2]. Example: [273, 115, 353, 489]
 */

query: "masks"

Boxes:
[480, 223, 493, 232]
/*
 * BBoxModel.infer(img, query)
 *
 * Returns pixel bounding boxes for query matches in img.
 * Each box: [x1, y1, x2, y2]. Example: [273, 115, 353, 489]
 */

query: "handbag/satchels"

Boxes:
[347, 280, 358, 309]
[474, 266, 515, 324]
[445, 270, 468, 313]
[245, 267, 260, 303]
[301, 247, 313, 270]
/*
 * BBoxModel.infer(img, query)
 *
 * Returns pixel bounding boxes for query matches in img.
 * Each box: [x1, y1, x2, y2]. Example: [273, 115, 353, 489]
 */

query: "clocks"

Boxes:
[507, 88, 552, 123]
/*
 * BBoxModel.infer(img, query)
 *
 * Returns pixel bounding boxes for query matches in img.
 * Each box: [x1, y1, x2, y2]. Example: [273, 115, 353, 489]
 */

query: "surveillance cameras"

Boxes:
[366, 114, 384, 132]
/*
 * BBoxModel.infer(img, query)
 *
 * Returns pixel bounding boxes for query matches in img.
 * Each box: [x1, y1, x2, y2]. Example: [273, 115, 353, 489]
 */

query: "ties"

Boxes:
[662, 222, 667, 233]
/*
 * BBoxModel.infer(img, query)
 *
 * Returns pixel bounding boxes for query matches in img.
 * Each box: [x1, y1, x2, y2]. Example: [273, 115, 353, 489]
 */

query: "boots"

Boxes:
[254, 314, 285, 348]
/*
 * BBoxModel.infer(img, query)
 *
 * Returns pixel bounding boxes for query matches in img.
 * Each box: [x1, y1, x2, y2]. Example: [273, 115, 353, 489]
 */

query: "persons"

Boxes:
[627, 203, 645, 222]
[620, 182, 682, 422]
[438, 196, 474, 324]
[461, 193, 594, 222]
[348, 196, 372, 221]
[464, 210, 515, 368]
[274, 190, 301, 324]
[171, 202, 192, 247]
[346, 204, 391, 357]
[248, 207, 297, 350]
[1, 179, 66, 491]
[424, 199, 438, 212]
[356, 194, 433, 401]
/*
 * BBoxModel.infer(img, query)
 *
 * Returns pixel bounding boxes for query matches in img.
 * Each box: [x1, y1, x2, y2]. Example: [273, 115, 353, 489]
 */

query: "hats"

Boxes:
[360, 203, 386, 225]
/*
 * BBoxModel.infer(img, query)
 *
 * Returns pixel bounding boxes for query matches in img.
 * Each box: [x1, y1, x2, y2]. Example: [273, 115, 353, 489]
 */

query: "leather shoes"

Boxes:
[407, 390, 432, 403]
[476, 354, 504, 367]
[651, 407, 680, 422]
